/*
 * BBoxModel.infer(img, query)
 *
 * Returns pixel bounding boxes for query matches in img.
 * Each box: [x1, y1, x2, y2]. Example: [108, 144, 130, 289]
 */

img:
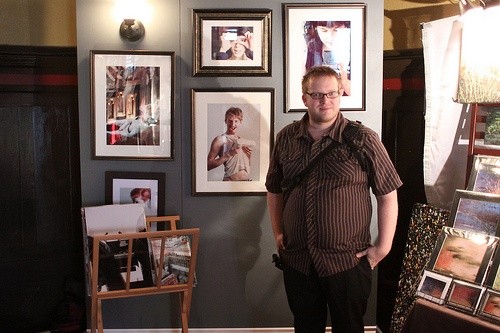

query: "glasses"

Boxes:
[306, 91, 340, 99]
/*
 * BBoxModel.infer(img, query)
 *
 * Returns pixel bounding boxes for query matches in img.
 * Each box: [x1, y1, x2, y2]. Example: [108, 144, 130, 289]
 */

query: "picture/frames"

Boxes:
[282, 2, 367, 114]
[89, 49, 176, 161]
[190, 87, 274, 197]
[412, 155, 500, 326]
[105, 171, 165, 232]
[191, 8, 273, 77]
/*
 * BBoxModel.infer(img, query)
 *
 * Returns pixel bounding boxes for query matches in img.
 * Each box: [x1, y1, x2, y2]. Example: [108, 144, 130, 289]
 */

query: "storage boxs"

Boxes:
[100, 238, 153, 291]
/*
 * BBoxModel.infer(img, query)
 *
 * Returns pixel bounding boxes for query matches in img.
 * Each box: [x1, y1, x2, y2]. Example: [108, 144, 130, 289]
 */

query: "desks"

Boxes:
[402, 300, 500, 333]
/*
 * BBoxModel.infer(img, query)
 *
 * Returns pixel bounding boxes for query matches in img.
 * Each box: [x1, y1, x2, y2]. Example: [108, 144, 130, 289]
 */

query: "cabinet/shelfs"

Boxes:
[377, 47, 428, 333]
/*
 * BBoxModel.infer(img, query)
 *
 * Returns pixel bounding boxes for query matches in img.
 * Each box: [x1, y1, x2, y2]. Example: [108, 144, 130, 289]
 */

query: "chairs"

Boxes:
[87, 215, 200, 333]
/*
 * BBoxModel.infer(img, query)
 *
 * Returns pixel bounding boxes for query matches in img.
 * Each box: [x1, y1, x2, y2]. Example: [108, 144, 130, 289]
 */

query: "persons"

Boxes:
[264, 66, 403, 333]
[207, 108, 253, 181]
[217, 27, 253, 60]
[304, 21, 350, 95]
